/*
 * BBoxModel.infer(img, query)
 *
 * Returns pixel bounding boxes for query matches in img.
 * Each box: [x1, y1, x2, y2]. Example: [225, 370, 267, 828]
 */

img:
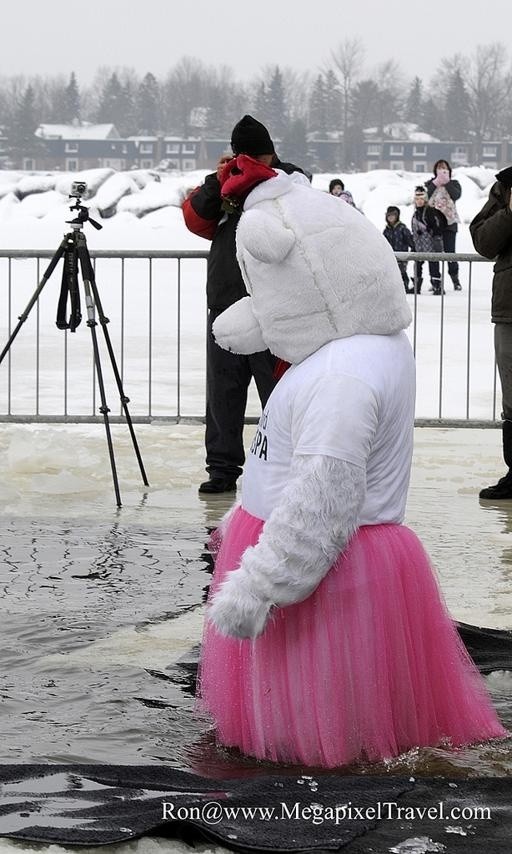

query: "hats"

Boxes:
[386, 206, 401, 223]
[412, 184, 428, 203]
[231, 113, 275, 156]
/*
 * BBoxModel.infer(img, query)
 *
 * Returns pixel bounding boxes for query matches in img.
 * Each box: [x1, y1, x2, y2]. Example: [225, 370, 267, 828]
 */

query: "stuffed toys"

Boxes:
[194, 151, 511, 769]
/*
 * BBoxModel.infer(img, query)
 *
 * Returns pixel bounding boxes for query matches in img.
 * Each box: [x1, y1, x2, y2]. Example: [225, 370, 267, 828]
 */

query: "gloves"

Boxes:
[432, 170, 449, 185]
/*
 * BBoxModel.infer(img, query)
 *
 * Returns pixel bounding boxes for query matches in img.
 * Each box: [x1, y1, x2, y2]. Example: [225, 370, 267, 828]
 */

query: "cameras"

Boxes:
[71, 182, 88, 196]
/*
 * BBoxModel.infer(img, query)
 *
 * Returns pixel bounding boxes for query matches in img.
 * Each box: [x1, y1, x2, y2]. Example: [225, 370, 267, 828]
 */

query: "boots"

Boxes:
[406, 273, 461, 294]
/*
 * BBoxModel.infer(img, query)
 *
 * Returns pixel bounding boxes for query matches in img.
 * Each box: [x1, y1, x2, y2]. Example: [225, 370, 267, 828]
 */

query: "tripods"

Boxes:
[0, 221, 151, 510]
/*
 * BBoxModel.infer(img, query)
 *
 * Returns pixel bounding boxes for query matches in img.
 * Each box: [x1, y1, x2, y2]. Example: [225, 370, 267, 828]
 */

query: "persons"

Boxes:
[383, 205, 416, 292]
[423, 158, 462, 291]
[406, 185, 445, 295]
[467, 164, 511, 500]
[329, 178, 354, 207]
[181, 112, 312, 494]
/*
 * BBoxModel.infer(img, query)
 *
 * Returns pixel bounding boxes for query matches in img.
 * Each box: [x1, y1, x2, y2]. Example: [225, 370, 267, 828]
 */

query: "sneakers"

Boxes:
[199, 476, 239, 493]
[477, 474, 512, 499]
